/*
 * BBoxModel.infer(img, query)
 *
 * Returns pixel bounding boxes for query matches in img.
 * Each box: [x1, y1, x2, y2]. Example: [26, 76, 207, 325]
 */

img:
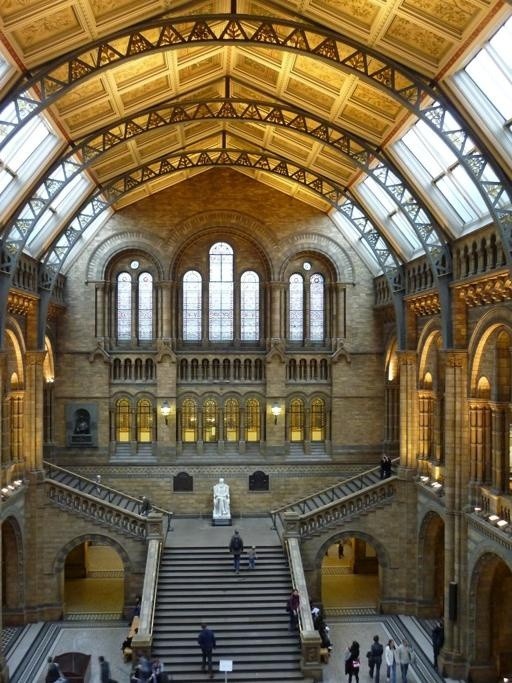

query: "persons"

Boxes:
[396, 639, 415, 682]
[213, 476, 231, 518]
[129, 653, 168, 683]
[98, 655, 109, 682]
[126, 594, 142, 623]
[47, 655, 65, 683]
[140, 493, 154, 516]
[385, 638, 398, 682]
[95, 474, 103, 495]
[431, 618, 445, 670]
[76, 412, 89, 438]
[367, 634, 383, 682]
[344, 641, 361, 682]
[381, 453, 392, 479]
[197, 622, 217, 682]
[310, 603, 333, 654]
[338, 542, 344, 560]
[248, 544, 259, 570]
[284, 588, 300, 632]
[229, 528, 244, 573]
[120, 627, 137, 654]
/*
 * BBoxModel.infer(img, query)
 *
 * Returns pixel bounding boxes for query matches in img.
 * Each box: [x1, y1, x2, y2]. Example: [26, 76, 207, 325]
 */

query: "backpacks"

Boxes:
[233, 536, 241, 549]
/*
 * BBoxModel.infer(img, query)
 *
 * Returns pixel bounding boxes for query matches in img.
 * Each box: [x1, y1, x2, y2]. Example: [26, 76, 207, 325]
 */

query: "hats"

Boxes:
[252, 546, 255, 549]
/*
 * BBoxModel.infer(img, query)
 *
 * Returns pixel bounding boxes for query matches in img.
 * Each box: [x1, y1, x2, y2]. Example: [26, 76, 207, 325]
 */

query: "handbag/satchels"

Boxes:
[352, 660, 360, 668]
[287, 600, 290, 611]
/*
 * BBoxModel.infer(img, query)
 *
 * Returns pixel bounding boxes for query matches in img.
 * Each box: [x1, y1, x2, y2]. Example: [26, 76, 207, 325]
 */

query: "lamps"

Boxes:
[161, 400, 170, 425]
[0, 480, 23, 498]
[271, 402, 281, 425]
[422, 475, 445, 498]
[475, 507, 509, 531]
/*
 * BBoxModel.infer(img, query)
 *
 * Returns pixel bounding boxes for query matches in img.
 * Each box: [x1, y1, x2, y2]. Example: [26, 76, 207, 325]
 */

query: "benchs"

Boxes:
[320, 648, 329, 664]
[124, 616, 139, 663]
